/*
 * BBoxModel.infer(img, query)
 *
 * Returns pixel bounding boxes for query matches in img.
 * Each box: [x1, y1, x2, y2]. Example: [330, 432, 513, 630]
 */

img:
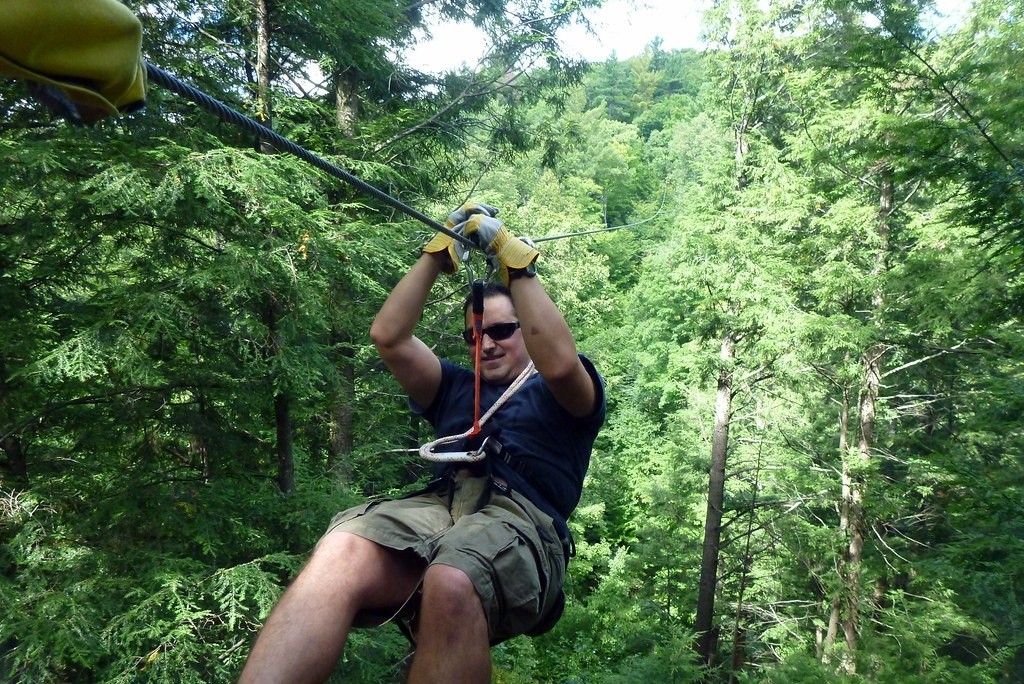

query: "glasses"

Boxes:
[462, 321, 521, 346]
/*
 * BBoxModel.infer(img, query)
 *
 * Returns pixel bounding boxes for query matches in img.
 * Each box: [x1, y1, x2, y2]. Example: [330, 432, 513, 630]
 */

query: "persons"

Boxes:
[235, 203, 606, 684]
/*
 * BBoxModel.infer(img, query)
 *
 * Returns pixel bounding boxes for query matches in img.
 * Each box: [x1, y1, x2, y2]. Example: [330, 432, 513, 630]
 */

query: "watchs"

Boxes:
[509, 260, 536, 279]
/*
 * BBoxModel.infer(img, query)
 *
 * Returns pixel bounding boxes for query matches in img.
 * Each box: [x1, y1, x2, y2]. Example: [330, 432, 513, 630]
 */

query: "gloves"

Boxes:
[451, 213, 540, 287]
[422, 203, 499, 274]
